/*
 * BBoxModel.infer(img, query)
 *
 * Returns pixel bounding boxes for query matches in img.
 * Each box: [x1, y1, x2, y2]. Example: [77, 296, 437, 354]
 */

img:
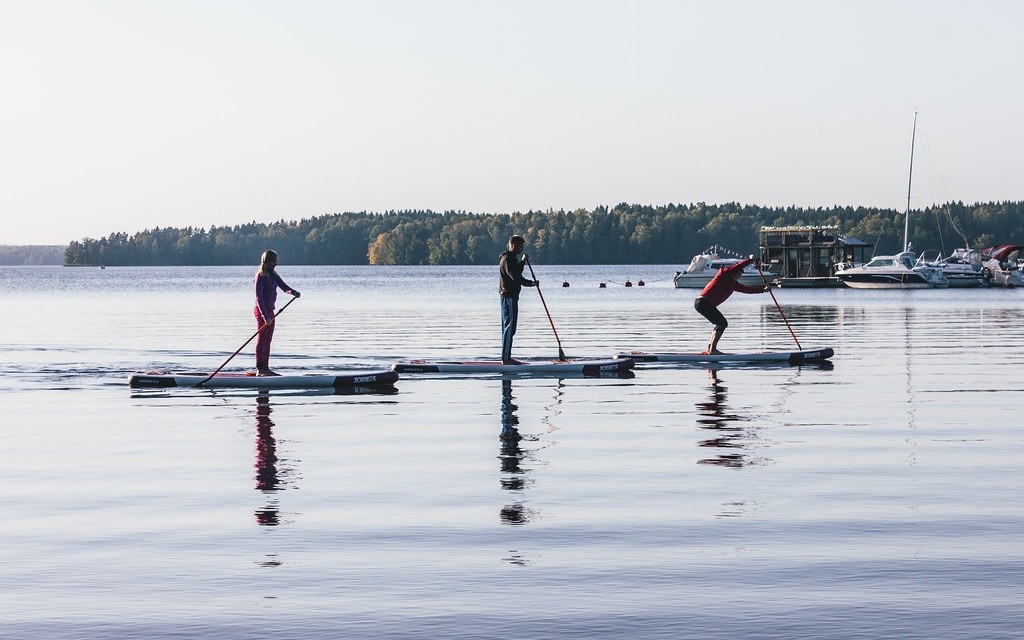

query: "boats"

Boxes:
[673, 253, 778, 289]
[924, 248, 995, 287]
[980, 244, 1024, 289]
[101, 264, 105, 269]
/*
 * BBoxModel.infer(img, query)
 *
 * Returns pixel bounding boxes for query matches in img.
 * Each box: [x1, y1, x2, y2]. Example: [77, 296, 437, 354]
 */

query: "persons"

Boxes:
[254, 250, 301, 377]
[694, 258, 771, 355]
[499, 236, 539, 365]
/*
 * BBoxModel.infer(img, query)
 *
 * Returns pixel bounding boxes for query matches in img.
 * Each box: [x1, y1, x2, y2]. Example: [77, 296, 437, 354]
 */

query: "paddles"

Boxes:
[524, 254, 567, 358]
[195, 294, 299, 389]
[753, 259, 801, 351]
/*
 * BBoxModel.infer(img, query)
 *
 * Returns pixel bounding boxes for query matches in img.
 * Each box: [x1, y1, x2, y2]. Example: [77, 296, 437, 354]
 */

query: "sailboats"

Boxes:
[835, 111, 950, 290]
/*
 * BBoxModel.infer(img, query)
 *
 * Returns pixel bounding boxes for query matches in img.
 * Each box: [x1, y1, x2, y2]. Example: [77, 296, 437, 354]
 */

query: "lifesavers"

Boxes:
[982, 267, 991, 280]
